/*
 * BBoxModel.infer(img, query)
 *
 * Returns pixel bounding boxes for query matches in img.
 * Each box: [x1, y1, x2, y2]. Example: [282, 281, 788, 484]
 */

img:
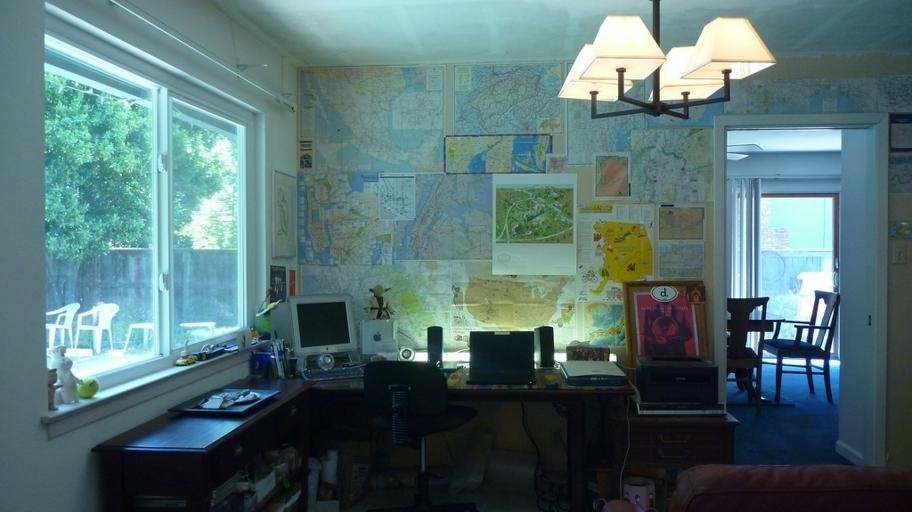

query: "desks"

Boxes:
[310, 360, 639, 512]
[725, 306, 787, 395]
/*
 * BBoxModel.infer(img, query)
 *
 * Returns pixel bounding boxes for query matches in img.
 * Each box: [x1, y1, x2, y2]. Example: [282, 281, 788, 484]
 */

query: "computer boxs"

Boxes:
[359, 318, 398, 356]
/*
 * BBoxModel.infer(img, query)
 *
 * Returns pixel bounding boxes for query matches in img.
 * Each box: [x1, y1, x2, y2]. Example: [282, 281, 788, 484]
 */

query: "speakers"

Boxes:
[399, 348, 415, 361]
[426, 325, 443, 367]
[534, 325, 555, 368]
[317, 353, 335, 370]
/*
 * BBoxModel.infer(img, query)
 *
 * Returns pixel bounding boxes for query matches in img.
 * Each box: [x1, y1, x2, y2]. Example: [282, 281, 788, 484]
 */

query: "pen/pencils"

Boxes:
[274, 329, 278, 339]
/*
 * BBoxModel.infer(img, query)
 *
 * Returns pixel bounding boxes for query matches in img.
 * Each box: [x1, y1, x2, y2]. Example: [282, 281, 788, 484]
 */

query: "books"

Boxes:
[129, 475, 238, 510]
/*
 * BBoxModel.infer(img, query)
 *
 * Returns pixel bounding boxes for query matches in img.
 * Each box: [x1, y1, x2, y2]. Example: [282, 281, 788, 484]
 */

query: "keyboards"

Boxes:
[302, 367, 364, 381]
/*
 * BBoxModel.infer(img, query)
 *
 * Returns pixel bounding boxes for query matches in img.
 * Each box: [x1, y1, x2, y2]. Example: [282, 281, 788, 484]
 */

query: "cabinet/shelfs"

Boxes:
[606, 410, 741, 484]
[87, 376, 314, 512]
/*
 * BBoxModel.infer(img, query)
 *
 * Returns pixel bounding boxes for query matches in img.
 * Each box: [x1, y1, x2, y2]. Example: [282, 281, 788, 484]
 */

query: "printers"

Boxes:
[635, 354, 718, 403]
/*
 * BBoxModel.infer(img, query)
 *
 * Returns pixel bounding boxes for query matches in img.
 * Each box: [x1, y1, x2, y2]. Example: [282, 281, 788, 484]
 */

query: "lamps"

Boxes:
[554, 0, 782, 121]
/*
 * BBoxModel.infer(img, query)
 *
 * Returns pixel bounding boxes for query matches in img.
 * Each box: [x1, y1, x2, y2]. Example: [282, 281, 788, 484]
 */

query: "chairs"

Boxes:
[757, 286, 843, 408]
[664, 459, 912, 511]
[353, 358, 488, 511]
[727, 294, 772, 412]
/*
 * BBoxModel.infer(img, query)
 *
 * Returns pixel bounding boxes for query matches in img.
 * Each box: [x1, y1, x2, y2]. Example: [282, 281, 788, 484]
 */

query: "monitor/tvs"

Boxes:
[270, 293, 357, 356]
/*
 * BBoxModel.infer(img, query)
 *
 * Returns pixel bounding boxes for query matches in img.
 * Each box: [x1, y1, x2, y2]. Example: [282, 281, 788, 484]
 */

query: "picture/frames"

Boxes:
[622, 278, 711, 389]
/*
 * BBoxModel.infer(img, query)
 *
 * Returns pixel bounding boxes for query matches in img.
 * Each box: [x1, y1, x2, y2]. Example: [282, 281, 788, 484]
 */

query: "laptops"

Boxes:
[465, 330, 537, 385]
